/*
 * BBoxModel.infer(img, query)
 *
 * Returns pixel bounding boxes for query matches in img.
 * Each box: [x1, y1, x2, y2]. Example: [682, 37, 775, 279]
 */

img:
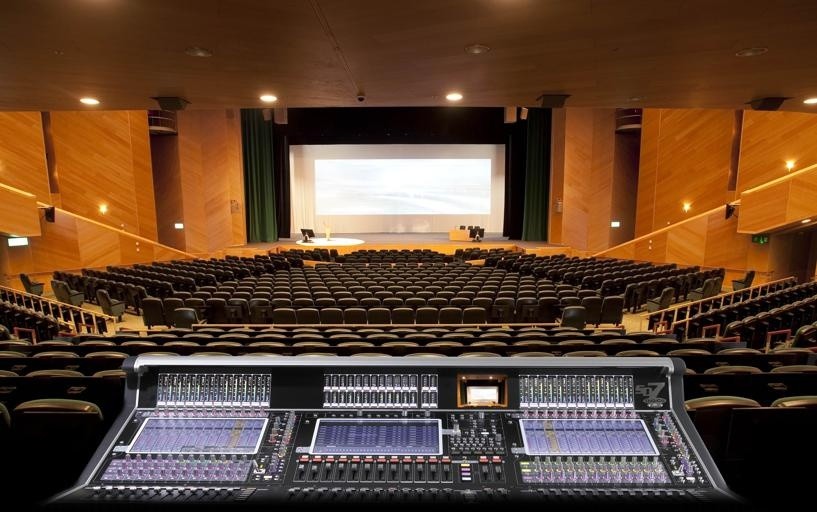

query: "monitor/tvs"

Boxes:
[470, 229, 484, 242]
[301, 229, 315, 242]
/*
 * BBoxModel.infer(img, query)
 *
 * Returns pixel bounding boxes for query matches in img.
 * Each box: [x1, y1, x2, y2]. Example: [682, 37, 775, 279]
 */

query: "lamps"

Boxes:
[37, 205, 56, 223]
[724, 203, 739, 220]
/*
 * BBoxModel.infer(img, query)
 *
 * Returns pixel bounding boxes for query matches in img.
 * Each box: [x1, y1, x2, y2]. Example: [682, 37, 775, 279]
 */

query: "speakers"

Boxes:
[725, 203, 733, 219]
[158, 98, 186, 111]
[45, 207, 55, 222]
[751, 97, 782, 111]
[541, 94, 566, 107]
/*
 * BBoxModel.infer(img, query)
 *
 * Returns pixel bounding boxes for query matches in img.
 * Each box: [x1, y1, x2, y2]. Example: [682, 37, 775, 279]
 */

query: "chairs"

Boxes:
[0, 248, 817, 512]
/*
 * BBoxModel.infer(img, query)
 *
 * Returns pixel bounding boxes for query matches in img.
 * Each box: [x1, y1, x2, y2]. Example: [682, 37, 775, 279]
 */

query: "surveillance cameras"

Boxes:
[357, 95, 365, 101]
